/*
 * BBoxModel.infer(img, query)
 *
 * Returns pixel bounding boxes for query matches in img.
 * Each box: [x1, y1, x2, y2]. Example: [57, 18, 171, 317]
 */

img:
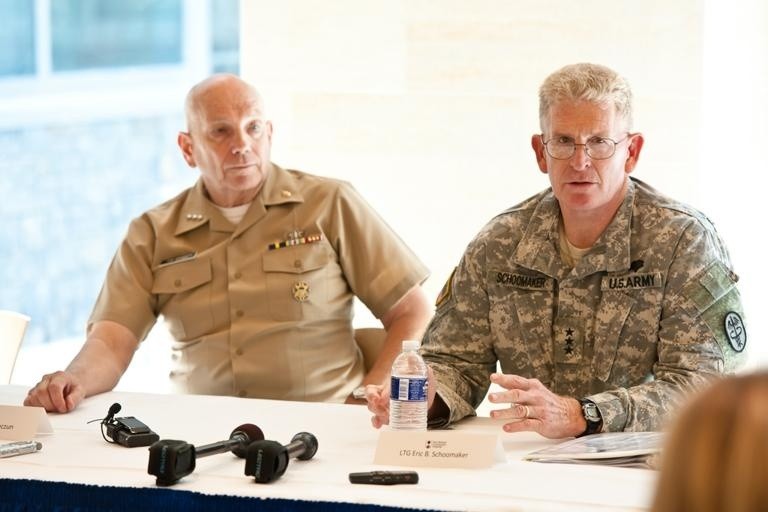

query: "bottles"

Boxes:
[388, 339, 428, 443]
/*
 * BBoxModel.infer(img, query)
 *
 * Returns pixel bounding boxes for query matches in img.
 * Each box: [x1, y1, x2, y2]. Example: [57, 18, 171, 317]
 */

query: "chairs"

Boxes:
[0, 310, 31, 384]
[354, 328, 387, 377]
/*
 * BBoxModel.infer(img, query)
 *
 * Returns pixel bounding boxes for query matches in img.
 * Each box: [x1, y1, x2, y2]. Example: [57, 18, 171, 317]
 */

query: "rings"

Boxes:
[523, 403, 531, 419]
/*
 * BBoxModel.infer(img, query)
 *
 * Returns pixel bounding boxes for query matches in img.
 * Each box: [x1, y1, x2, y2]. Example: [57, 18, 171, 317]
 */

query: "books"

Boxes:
[524, 429, 673, 460]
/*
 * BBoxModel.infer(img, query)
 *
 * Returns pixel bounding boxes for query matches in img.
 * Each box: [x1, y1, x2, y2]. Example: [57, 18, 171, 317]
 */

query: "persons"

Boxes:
[647, 373, 768, 511]
[364, 62, 748, 440]
[21, 71, 433, 413]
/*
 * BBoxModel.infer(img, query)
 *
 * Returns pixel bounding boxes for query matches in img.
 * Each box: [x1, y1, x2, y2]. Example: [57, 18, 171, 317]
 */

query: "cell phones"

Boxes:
[349, 470, 420, 486]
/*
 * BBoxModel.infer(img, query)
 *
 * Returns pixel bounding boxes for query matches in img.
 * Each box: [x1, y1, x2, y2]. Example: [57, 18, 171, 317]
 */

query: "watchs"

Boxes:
[575, 397, 602, 438]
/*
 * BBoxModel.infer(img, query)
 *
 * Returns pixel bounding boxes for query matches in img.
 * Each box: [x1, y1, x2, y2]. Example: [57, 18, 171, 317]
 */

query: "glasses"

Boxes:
[539, 133, 630, 160]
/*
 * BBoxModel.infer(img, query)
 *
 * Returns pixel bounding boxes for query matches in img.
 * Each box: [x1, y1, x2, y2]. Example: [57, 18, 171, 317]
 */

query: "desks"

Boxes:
[0, 382, 667, 512]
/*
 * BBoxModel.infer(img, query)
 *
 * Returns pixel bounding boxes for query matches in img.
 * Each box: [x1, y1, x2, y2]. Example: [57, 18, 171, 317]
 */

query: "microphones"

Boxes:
[149, 423, 264, 488]
[105, 402, 122, 419]
[244, 431, 318, 484]
